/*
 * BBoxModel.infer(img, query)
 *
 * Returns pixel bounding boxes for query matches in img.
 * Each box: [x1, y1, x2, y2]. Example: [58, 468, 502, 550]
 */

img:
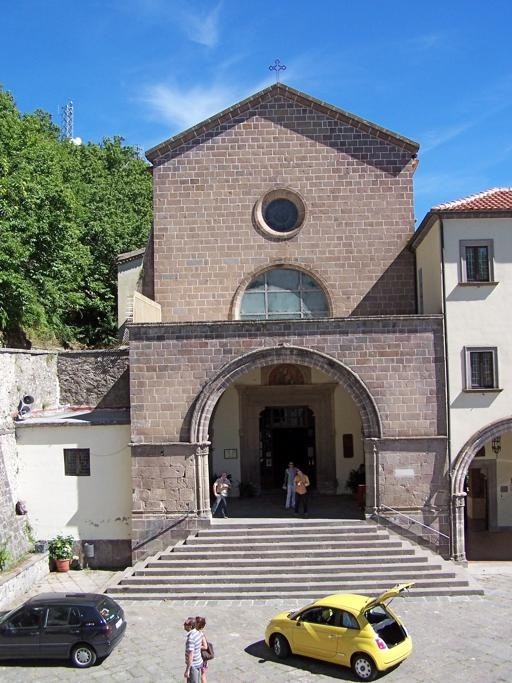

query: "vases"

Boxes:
[55, 558, 69, 571]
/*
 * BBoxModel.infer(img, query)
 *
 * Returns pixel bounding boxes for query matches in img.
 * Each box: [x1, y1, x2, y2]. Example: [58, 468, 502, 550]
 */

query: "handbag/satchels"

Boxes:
[201, 643, 214, 660]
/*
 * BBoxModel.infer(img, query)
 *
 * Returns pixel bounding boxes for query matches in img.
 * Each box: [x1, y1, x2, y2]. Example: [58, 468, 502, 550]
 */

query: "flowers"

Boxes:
[48, 535, 74, 559]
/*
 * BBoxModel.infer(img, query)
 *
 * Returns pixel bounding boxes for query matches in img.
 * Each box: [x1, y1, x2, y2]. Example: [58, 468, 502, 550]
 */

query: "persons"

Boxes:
[195, 616, 208, 683]
[211, 472, 232, 518]
[293, 467, 310, 516]
[183, 616, 203, 683]
[282, 461, 297, 510]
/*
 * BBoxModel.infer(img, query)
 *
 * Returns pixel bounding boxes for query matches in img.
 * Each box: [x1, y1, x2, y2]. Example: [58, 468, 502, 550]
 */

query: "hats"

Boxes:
[221, 490, 230, 497]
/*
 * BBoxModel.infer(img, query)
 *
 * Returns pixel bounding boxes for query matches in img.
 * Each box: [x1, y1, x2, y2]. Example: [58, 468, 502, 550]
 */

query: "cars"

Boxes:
[0, 592, 127, 668]
[264, 582, 415, 682]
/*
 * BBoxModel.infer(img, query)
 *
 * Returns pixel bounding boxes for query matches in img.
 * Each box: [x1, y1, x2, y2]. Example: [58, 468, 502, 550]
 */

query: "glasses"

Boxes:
[184, 622, 191, 626]
[288, 463, 294, 465]
